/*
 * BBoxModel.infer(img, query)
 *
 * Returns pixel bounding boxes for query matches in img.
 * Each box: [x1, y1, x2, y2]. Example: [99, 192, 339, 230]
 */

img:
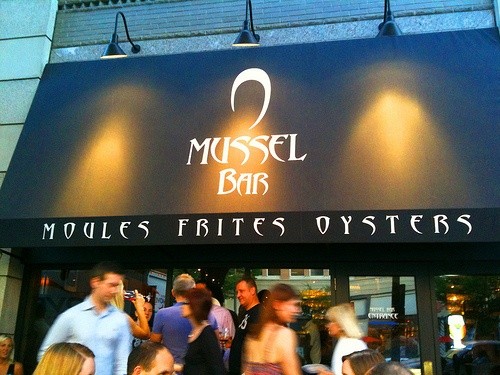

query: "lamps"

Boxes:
[232, 0, 260, 46]
[375, 0, 404, 38]
[100, 10, 141, 59]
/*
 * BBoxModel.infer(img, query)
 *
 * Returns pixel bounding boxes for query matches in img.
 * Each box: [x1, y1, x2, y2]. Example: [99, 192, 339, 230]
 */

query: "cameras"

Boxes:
[123, 290, 135, 298]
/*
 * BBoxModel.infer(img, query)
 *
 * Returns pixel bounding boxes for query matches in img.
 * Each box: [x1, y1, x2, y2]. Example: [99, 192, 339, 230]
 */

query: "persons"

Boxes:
[197, 280, 235, 371]
[288, 313, 322, 375]
[111, 280, 151, 339]
[127, 340, 174, 375]
[127, 300, 154, 348]
[318, 303, 368, 375]
[229, 277, 269, 375]
[243, 284, 302, 375]
[151, 273, 198, 375]
[33, 342, 95, 375]
[210, 285, 240, 375]
[179, 287, 225, 375]
[341, 349, 386, 375]
[369, 361, 414, 375]
[34, 260, 132, 375]
[0, 335, 24, 375]
